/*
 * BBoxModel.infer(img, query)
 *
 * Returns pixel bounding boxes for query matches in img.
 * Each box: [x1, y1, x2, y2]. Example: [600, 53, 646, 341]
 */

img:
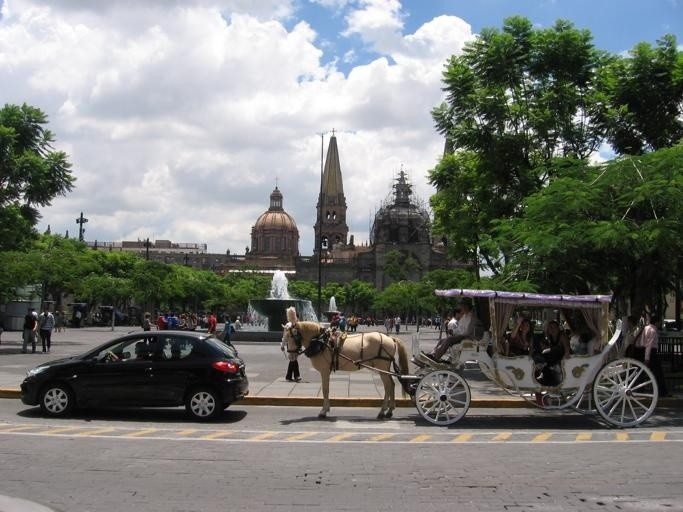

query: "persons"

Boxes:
[330, 310, 459, 335]
[246, 311, 268, 328]
[54, 306, 144, 333]
[280, 307, 304, 383]
[144, 311, 241, 355]
[112, 342, 150, 363]
[426, 297, 662, 396]
[20, 307, 55, 354]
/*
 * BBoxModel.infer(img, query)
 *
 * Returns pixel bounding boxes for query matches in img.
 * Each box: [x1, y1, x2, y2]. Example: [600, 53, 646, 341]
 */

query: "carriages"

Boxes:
[279, 287, 660, 431]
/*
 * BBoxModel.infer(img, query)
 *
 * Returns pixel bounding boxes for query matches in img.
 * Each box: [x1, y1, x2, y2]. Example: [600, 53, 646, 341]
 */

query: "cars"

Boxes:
[19, 330, 249, 421]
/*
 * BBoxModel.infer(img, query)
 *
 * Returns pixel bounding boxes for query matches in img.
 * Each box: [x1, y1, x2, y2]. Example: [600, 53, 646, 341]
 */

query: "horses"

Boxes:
[279, 322, 409, 419]
